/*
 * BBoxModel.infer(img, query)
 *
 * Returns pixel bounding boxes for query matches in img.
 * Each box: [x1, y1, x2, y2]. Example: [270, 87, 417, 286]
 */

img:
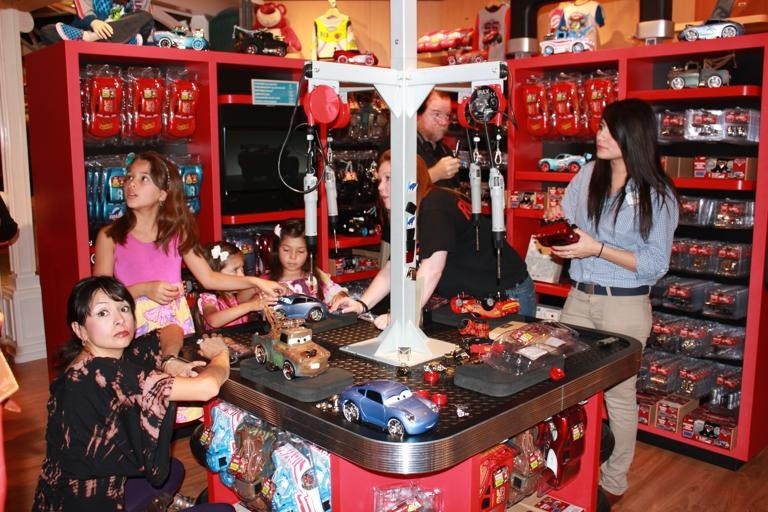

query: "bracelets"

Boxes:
[597, 242, 604, 257]
[160, 355, 175, 373]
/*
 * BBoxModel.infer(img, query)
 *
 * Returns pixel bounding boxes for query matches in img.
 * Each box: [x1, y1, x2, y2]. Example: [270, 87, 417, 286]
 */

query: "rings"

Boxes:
[195, 338, 203, 345]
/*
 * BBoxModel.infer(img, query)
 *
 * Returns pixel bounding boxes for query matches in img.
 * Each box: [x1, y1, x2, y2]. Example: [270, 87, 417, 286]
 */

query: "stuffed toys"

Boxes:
[254, 4, 300, 59]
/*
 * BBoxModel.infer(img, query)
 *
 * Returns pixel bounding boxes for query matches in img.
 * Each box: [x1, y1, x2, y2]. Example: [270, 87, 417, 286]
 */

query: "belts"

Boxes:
[570, 280, 649, 296]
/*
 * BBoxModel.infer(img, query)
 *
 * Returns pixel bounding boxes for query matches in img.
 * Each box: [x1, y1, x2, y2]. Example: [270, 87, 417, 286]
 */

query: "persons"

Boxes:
[38, 0, 195, 46]
[94, 152, 287, 339]
[258, 218, 350, 311]
[193, 242, 280, 331]
[330, 150, 536, 330]
[378, 92, 462, 272]
[555, 0, 605, 50]
[546, 0, 572, 34]
[33, 276, 234, 511]
[537, 97, 679, 506]
